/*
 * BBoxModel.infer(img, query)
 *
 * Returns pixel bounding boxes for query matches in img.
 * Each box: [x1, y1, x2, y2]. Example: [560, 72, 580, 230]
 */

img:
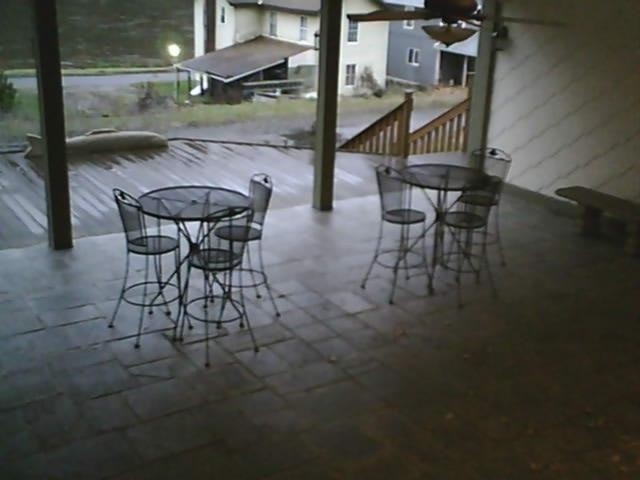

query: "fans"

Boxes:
[345, 0, 569, 49]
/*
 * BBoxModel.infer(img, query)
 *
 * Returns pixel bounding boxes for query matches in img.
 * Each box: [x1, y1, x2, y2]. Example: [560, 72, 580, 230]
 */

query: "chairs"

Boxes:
[110, 169, 280, 370]
[364, 150, 510, 302]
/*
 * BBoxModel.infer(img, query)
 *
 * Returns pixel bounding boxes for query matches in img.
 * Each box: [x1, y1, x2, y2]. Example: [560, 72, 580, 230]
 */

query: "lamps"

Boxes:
[419, 17, 477, 48]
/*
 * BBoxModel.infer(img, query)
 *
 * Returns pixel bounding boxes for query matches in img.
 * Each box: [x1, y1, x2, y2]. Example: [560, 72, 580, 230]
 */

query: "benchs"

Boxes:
[552, 184, 640, 257]
